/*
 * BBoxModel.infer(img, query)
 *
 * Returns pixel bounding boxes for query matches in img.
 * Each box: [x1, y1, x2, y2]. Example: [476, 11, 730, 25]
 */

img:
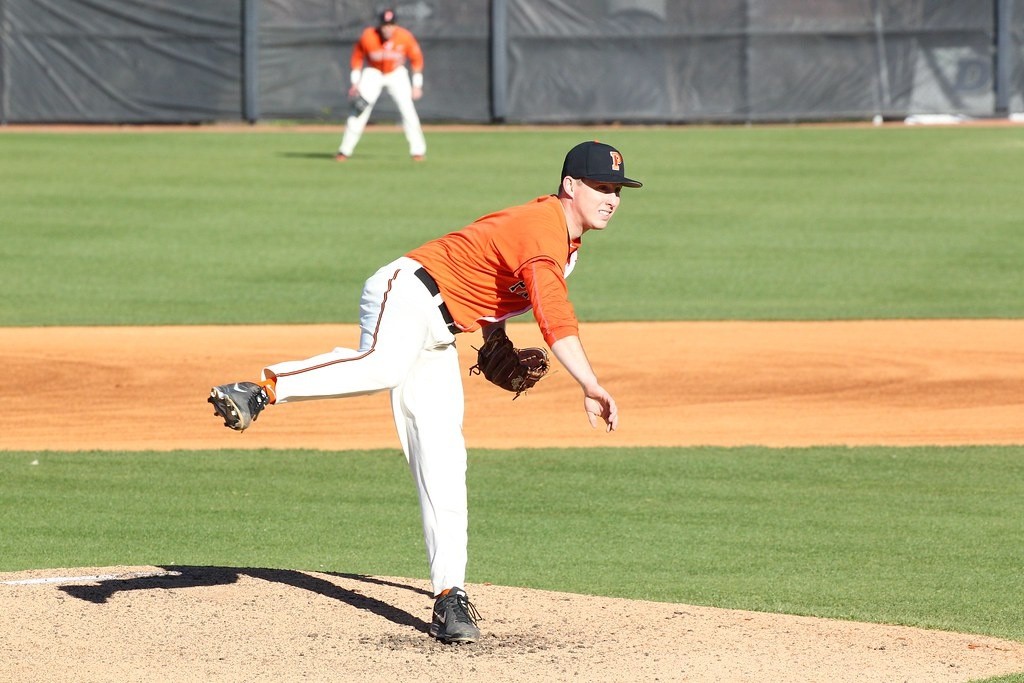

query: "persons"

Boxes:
[332, 8, 429, 166]
[207, 140, 642, 637]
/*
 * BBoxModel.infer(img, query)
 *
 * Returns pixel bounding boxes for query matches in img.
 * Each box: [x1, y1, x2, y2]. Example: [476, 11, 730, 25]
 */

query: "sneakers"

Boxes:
[207, 381, 269, 429]
[430, 586, 480, 642]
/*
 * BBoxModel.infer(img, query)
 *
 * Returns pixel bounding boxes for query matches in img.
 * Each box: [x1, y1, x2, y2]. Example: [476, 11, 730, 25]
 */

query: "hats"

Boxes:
[380, 10, 396, 25]
[562, 141, 643, 188]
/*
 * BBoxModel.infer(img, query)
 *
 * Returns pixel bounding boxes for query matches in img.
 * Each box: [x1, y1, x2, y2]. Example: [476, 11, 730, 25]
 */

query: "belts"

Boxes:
[414, 267, 462, 336]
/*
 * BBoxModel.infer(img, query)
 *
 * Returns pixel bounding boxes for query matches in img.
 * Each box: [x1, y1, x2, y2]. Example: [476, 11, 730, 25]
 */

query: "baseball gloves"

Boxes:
[469, 328, 551, 404]
[348, 93, 369, 113]
